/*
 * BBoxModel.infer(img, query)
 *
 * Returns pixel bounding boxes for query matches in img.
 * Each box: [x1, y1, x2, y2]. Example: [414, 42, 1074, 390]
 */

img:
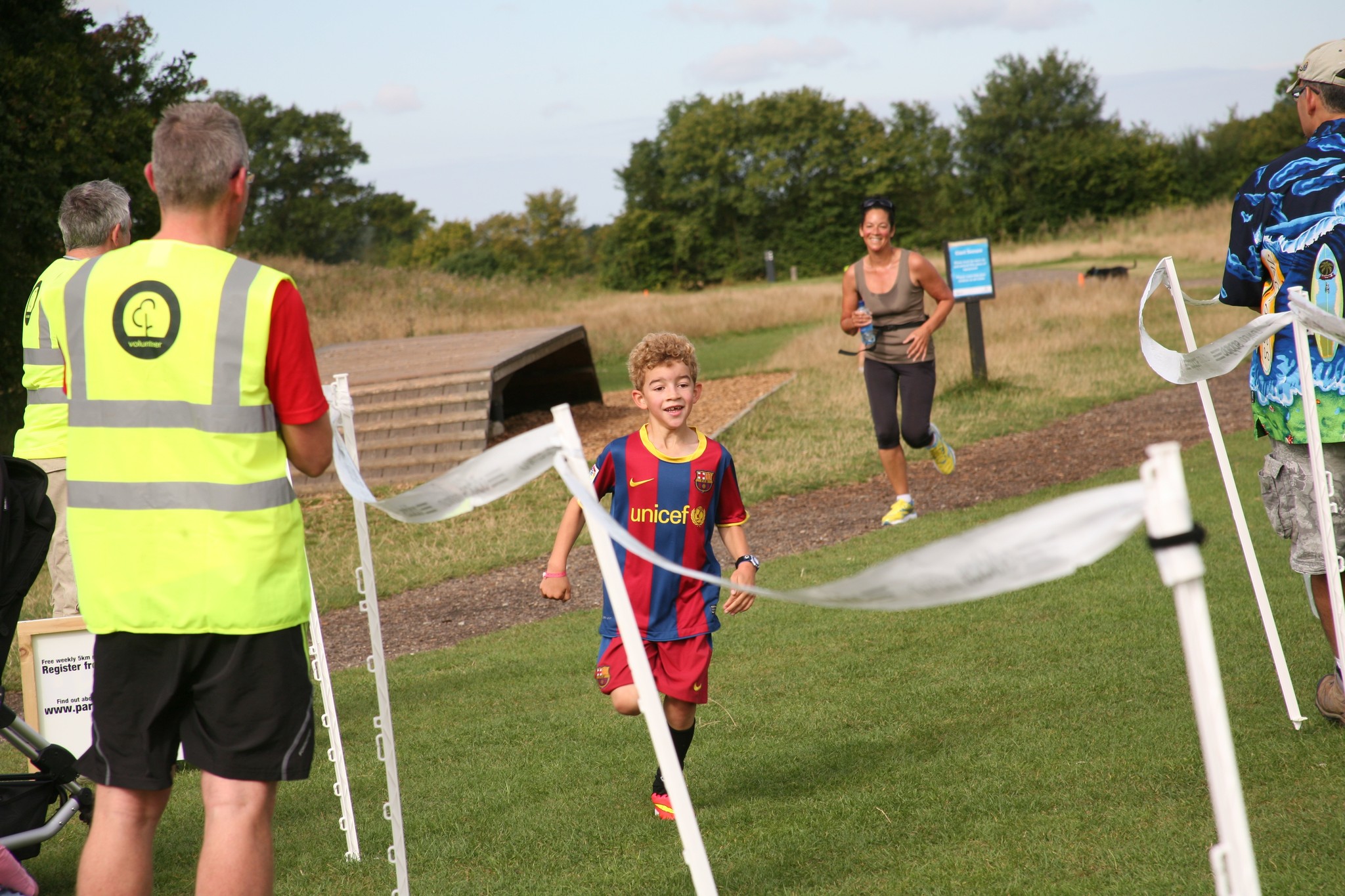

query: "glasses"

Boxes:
[863, 199, 892, 208]
[231, 170, 255, 183]
[1292, 86, 1319, 102]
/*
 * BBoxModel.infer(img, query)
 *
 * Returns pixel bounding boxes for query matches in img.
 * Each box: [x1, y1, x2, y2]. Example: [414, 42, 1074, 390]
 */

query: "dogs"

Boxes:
[1082, 258, 1139, 284]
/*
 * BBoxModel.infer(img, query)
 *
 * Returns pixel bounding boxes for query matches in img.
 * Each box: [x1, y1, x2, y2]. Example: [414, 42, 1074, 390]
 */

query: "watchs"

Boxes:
[734, 555, 761, 573]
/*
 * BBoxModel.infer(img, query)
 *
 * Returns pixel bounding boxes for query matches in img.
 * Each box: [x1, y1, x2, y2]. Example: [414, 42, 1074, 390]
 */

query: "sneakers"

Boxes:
[881, 499, 917, 526]
[651, 792, 675, 820]
[1316, 674, 1345, 726]
[925, 423, 956, 475]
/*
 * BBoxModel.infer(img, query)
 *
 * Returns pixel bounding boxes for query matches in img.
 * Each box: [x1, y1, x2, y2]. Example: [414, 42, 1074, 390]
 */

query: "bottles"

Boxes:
[856, 300, 875, 344]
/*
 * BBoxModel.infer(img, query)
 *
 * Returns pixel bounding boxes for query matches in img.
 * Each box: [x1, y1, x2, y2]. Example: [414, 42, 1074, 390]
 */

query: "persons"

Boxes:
[1219, 40, 1345, 725]
[40, 101, 334, 896]
[538, 332, 758, 820]
[11, 179, 133, 616]
[840, 200, 955, 526]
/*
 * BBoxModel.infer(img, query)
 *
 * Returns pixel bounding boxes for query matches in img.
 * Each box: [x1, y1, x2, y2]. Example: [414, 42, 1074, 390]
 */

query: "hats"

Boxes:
[1286, 38, 1345, 94]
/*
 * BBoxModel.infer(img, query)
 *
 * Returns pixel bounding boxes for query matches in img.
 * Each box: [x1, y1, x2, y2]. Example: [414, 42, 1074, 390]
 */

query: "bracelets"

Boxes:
[542, 571, 568, 579]
